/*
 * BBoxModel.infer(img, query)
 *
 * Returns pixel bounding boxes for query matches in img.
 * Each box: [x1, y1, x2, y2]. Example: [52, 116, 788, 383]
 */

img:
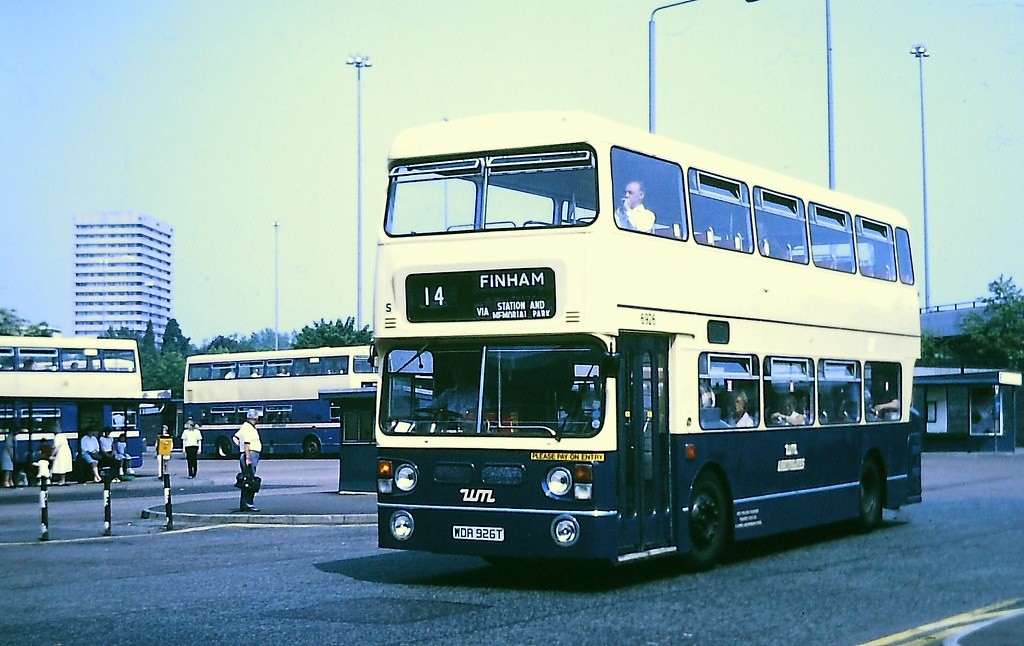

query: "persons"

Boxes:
[67, 362, 79, 371]
[770, 395, 806, 426]
[99, 425, 121, 483]
[50, 426, 72, 485]
[1, 357, 14, 371]
[32, 438, 55, 486]
[20, 359, 34, 370]
[327, 369, 344, 375]
[277, 367, 290, 376]
[426, 360, 496, 433]
[80, 426, 103, 483]
[224, 369, 235, 379]
[846, 373, 900, 422]
[613, 181, 655, 232]
[721, 391, 753, 428]
[154, 425, 173, 481]
[48, 357, 59, 371]
[115, 434, 135, 475]
[1, 426, 20, 487]
[11, 456, 29, 487]
[232, 409, 262, 511]
[557, 392, 593, 433]
[181, 418, 202, 478]
[251, 369, 262, 377]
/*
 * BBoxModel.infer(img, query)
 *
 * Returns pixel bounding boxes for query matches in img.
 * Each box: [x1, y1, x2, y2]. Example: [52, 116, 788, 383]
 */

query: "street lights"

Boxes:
[346, 53, 375, 331]
[273, 222, 280, 350]
[910, 45, 929, 313]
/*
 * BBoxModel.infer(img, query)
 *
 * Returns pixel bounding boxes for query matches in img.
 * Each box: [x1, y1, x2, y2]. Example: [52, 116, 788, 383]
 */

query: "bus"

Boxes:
[184, 344, 434, 459]
[372, 114, 922, 574]
[0, 335, 144, 481]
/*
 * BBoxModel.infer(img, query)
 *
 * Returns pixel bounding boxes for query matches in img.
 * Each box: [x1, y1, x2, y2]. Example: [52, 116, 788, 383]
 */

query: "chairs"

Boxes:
[655, 225, 917, 287]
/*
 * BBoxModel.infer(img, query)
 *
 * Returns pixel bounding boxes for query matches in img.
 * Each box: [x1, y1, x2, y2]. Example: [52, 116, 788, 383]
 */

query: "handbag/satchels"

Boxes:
[234, 463, 261, 493]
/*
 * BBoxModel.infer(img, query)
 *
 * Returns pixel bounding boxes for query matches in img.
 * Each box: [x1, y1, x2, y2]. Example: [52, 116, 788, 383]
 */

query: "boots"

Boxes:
[58, 475, 65, 485]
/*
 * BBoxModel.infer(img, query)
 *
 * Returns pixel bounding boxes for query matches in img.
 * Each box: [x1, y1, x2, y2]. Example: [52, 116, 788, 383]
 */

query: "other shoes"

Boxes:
[127, 468, 135, 474]
[187, 475, 192, 479]
[4, 481, 9, 487]
[116, 477, 120, 483]
[46, 479, 51, 485]
[9, 480, 14, 486]
[112, 479, 116, 482]
[240, 505, 261, 512]
[36, 479, 42, 486]
[94, 476, 102, 482]
[119, 466, 124, 475]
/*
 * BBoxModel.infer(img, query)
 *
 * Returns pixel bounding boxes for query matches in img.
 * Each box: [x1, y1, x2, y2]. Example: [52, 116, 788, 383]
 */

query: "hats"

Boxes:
[247, 409, 259, 419]
